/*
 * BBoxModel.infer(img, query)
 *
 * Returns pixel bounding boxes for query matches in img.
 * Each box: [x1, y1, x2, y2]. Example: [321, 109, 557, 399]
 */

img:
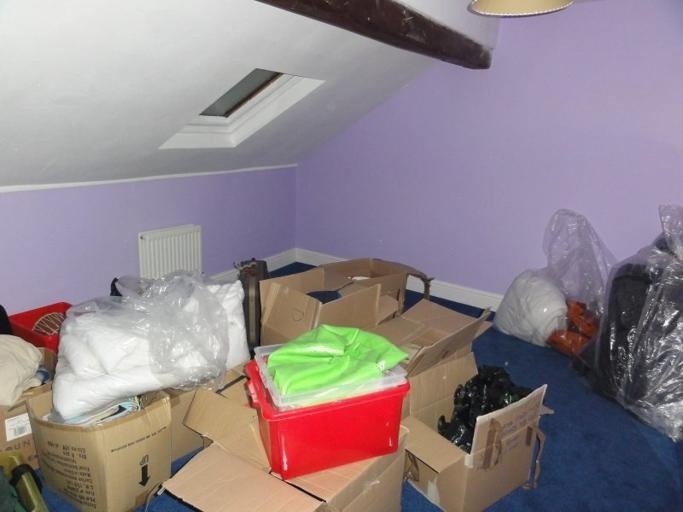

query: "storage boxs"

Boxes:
[1, 252, 548, 512]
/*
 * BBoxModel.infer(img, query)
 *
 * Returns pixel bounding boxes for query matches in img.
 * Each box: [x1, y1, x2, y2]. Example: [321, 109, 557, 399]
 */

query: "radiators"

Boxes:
[140, 224, 204, 284]
